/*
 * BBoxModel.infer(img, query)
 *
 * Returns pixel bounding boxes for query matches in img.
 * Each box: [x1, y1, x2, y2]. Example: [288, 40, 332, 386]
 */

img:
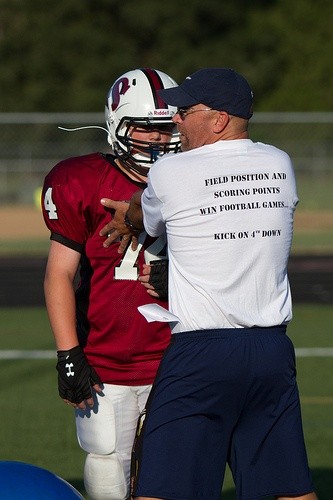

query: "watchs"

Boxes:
[125, 212, 145, 233]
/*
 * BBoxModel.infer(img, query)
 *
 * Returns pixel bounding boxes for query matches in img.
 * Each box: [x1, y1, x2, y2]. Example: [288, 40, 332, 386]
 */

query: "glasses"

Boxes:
[176, 107, 214, 121]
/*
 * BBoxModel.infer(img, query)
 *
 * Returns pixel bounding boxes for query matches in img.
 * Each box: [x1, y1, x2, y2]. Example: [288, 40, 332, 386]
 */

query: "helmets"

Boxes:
[104, 68, 183, 177]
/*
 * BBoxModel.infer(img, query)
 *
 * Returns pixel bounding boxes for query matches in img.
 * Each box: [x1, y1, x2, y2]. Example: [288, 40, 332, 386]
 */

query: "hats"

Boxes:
[158, 68, 254, 120]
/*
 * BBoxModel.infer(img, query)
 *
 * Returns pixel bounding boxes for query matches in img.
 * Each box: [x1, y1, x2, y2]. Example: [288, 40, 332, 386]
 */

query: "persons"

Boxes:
[41, 67, 180, 500]
[99, 68, 319, 500]
